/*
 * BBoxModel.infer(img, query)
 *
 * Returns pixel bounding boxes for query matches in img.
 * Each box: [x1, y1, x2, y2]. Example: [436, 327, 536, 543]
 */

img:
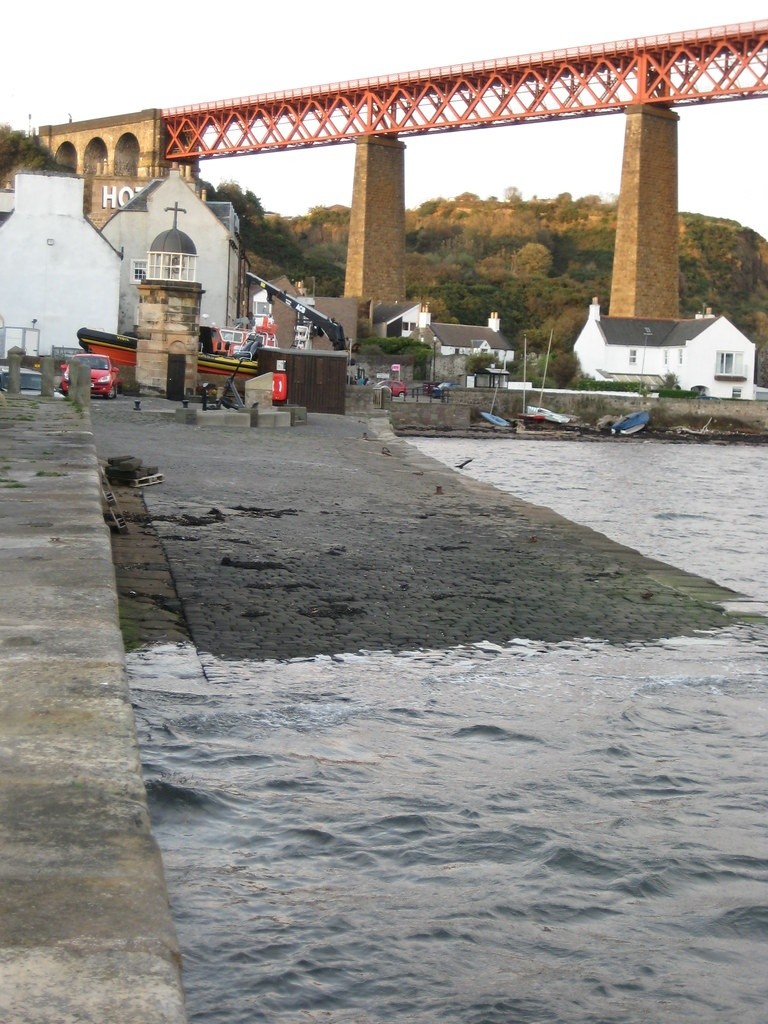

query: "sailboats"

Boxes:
[480, 349, 512, 428]
[526, 327, 570, 424]
[518, 334, 544, 426]
[611, 327, 654, 436]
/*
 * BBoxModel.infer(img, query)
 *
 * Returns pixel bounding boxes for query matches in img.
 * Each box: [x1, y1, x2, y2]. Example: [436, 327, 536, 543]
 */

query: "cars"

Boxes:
[56, 353, 121, 399]
[0, 365, 65, 401]
[375, 380, 407, 398]
[423, 380, 441, 395]
[431, 381, 462, 400]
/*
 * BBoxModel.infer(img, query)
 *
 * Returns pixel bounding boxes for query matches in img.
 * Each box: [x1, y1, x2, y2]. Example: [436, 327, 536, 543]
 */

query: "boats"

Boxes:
[76, 320, 289, 404]
